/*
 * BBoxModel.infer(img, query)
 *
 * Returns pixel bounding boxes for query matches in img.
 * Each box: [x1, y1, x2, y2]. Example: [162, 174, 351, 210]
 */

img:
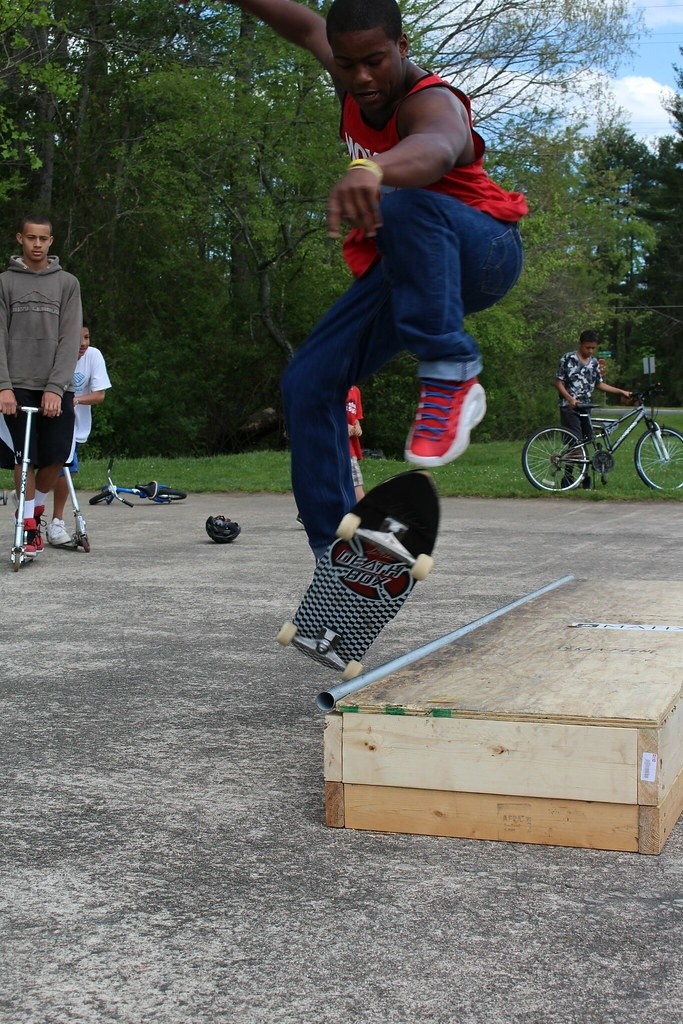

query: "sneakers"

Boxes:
[45, 517, 71, 545]
[22, 519, 38, 558]
[15, 505, 45, 553]
[404, 373, 487, 468]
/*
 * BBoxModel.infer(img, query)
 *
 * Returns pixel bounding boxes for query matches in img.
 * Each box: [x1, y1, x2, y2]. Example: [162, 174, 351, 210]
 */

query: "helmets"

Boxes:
[206, 516, 241, 543]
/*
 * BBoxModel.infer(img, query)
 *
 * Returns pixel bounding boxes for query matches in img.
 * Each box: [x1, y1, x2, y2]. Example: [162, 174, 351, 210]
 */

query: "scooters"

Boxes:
[42, 398, 91, 553]
[0, 404, 70, 573]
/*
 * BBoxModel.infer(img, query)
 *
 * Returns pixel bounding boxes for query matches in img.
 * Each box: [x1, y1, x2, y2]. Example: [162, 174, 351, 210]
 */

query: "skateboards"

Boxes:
[272, 467, 442, 684]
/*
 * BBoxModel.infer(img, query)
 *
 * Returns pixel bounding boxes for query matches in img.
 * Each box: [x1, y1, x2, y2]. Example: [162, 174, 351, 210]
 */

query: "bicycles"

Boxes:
[520, 382, 683, 498]
[90, 457, 187, 508]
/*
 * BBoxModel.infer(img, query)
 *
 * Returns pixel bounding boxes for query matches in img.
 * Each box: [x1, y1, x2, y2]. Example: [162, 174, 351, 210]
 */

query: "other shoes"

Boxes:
[581, 475, 594, 491]
[561, 475, 583, 490]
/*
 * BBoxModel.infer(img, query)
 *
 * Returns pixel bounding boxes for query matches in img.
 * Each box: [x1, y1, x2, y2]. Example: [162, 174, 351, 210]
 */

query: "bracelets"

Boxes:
[346, 159, 383, 183]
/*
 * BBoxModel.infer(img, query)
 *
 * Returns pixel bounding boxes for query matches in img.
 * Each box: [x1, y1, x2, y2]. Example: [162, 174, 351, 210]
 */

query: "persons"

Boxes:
[555, 330, 633, 489]
[0, 210, 83, 556]
[11, 324, 112, 544]
[344, 384, 366, 502]
[227, 0, 530, 563]
